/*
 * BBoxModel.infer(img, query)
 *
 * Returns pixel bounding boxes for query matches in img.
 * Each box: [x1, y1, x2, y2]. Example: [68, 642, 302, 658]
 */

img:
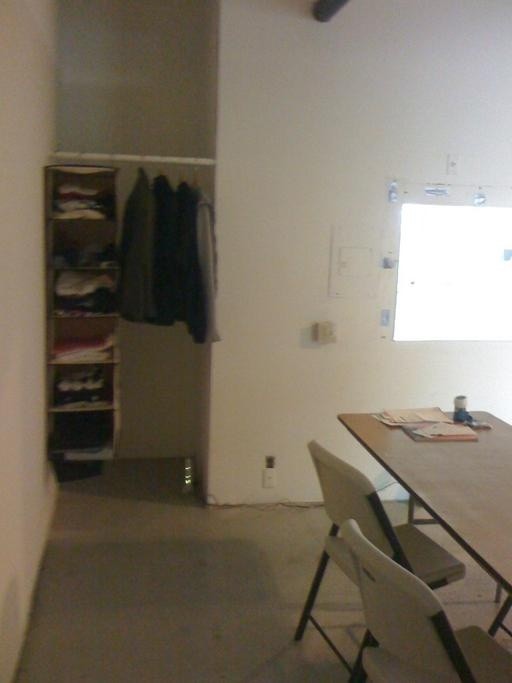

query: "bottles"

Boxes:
[454, 395, 467, 425]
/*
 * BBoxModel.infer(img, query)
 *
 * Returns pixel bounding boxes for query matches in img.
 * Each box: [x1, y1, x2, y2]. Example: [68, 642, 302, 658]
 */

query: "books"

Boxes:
[403, 423, 479, 442]
[372, 409, 452, 426]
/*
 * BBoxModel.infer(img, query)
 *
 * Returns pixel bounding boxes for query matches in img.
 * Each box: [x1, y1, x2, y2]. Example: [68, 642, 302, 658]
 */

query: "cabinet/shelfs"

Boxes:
[43, 165, 122, 464]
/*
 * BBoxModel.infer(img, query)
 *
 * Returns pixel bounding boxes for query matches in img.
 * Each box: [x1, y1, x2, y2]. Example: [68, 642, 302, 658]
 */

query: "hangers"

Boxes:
[137, 154, 145, 177]
[156, 156, 166, 187]
[192, 160, 204, 194]
[179, 158, 189, 187]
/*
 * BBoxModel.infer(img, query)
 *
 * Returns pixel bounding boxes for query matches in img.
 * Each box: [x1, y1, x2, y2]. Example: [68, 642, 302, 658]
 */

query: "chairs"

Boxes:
[293, 441, 466, 683]
[340, 519, 512, 683]
